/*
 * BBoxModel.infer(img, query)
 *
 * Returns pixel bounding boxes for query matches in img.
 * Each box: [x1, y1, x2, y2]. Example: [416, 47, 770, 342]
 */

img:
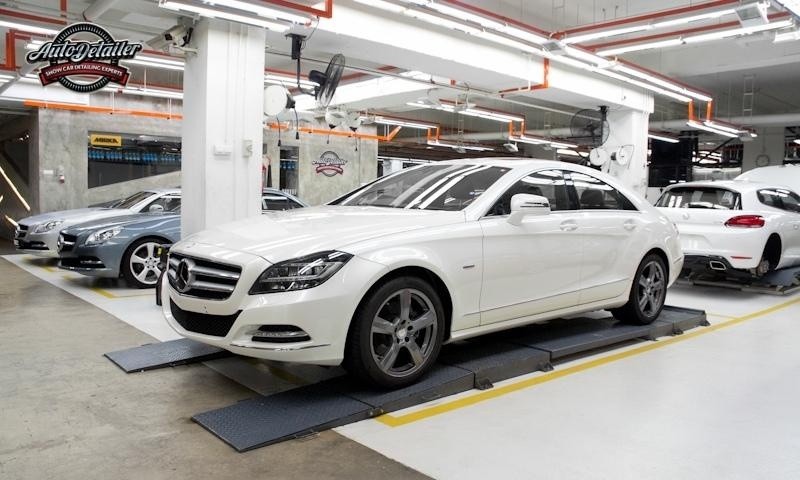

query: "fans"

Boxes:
[568, 105, 610, 151]
[284, 32, 347, 109]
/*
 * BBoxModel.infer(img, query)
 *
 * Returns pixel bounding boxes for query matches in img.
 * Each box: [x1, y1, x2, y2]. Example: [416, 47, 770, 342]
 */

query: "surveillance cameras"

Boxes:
[144, 32, 171, 52]
[162, 25, 187, 44]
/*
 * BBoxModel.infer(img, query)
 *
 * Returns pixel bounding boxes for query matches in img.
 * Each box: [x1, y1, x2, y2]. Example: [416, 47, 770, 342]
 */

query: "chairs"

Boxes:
[501, 187, 606, 214]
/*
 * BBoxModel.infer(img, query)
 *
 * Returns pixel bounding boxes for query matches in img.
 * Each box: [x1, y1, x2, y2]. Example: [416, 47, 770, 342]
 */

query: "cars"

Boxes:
[56, 187, 314, 289]
[650, 161, 800, 284]
[161, 157, 685, 393]
[14, 186, 182, 259]
